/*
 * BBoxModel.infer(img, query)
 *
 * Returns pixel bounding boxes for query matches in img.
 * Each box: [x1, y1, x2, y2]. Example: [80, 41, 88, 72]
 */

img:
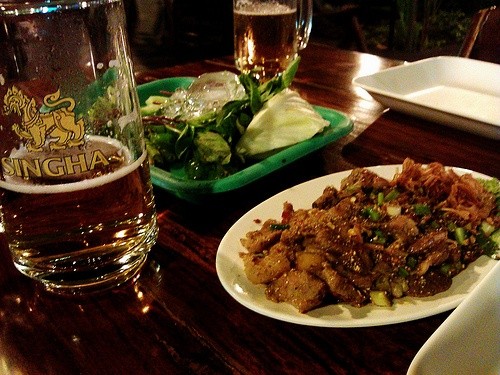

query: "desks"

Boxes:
[1, 41, 500, 375]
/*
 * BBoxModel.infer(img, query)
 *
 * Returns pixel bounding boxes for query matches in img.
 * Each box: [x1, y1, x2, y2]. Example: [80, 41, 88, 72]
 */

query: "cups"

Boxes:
[0, 0, 158, 295]
[233, 0, 299, 84]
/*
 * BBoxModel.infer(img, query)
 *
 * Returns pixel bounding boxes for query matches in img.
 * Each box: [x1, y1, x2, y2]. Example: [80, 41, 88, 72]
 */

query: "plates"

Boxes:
[136, 77, 354, 201]
[406, 259, 500, 375]
[352, 55, 500, 141]
[215, 164, 500, 328]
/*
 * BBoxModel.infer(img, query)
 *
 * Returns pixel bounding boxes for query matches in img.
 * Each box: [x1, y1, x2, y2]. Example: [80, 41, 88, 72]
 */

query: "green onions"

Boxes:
[267, 176, 500, 307]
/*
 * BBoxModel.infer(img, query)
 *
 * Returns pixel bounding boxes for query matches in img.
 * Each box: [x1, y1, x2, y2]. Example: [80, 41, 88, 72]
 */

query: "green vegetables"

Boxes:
[97, 54, 301, 180]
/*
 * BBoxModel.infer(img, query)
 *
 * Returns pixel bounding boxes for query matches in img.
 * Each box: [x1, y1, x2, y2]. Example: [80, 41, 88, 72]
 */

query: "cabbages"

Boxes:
[195, 88, 330, 163]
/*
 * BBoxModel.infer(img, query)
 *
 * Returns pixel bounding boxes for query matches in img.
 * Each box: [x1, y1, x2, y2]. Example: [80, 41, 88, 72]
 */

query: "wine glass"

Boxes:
[267, 0, 313, 99]
